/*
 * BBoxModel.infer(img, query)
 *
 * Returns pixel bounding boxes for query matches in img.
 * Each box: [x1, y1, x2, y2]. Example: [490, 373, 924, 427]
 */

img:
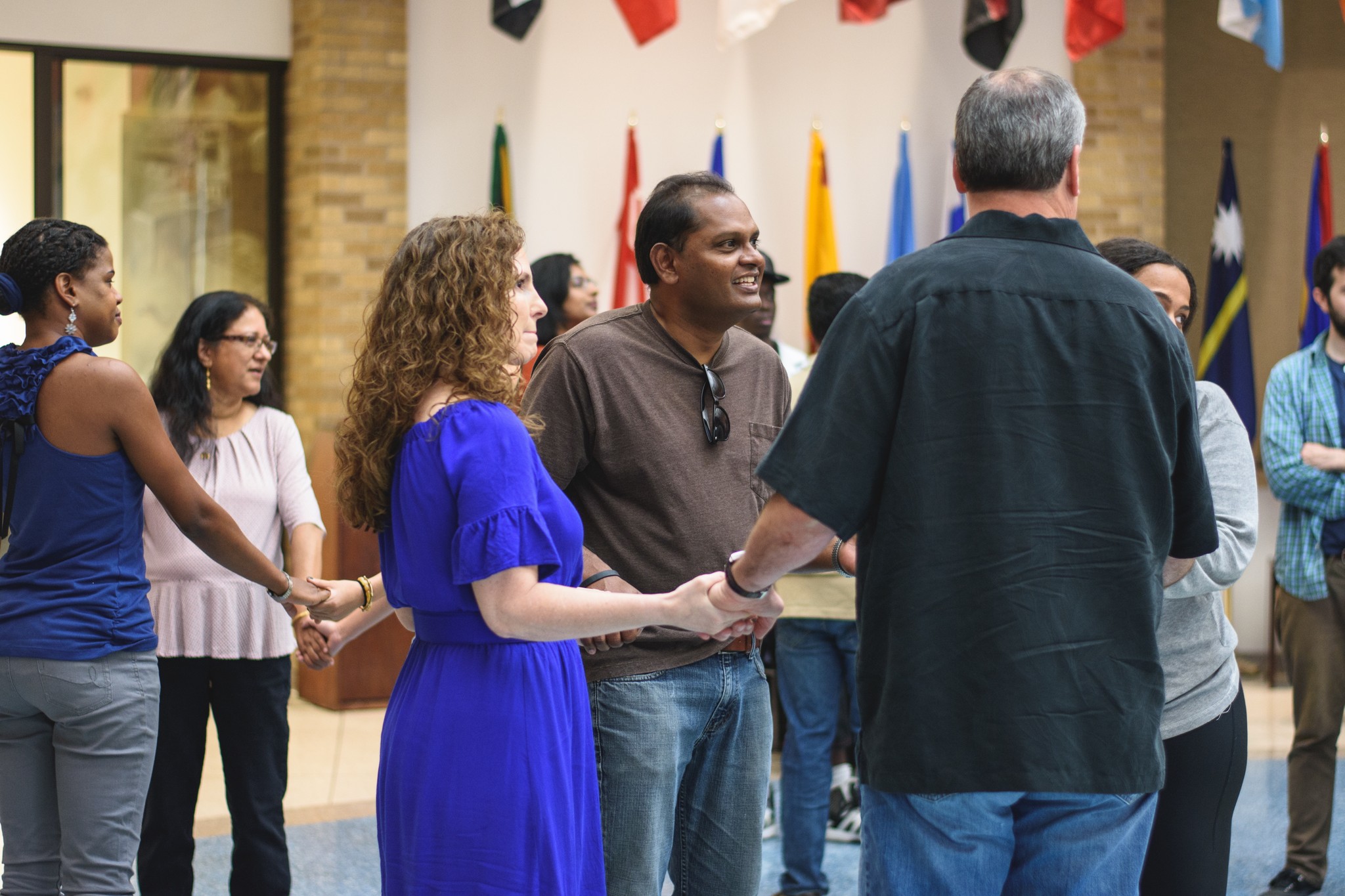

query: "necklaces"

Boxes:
[211, 399, 243, 420]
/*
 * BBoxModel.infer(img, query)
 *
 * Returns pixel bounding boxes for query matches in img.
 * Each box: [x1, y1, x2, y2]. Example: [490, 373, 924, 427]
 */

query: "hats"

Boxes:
[757, 248, 790, 283]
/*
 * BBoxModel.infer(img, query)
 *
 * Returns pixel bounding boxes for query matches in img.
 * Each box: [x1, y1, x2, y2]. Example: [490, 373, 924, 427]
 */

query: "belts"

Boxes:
[726, 633, 762, 654]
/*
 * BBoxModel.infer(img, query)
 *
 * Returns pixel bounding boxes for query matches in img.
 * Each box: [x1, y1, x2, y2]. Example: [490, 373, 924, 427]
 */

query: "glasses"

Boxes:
[700, 363, 731, 446]
[217, 332, 277, 355]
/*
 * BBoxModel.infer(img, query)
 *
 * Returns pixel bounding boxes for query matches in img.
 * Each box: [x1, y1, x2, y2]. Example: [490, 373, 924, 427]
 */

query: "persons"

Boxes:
[0, 66, 1345, 896]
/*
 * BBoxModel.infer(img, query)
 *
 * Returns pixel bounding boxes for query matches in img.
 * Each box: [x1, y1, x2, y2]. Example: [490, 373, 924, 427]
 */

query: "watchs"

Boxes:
[724, 549, 772, 599]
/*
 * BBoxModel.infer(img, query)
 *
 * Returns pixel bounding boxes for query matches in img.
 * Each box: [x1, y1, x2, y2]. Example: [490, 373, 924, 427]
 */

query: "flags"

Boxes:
[804, 127, 840, 353]
[836, 0, 904, 26]
[711, 136, 723, 177]
[941, 139, 967, 242]
[1298, 144, 1335, 351]
[613, 0, 677, 45]
[961, 0, 1023, 70]
[1217, 0, 1284, 74]
[1196, 135, 1256, 449]
[492, 0, 543, 39]
[884, 133, 916, 264]
[491, 124, 512, 218]
[611, 128, 646, 310]
[718, 0, 793, 40]
[1063, 0, 1127, 63]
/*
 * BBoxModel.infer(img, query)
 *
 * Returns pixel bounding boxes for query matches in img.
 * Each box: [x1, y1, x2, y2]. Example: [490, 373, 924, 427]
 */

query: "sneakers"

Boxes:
[825, 806, 862, 842]
[762, 807, 779, 839]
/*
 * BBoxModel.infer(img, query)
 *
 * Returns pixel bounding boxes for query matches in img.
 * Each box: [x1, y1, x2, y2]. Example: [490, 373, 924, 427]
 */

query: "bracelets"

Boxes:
[291, 610, 310, 639]
[579, 570, 621, 588]
[832, 537, 855, 578]
[266, 571, 293, 601]
[357, 575, 371, 612]
[363, 575, 374, 611]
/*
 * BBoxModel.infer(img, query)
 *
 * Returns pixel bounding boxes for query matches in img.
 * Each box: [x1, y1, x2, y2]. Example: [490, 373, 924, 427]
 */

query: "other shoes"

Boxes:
[1261, 870, 1320, 896]
